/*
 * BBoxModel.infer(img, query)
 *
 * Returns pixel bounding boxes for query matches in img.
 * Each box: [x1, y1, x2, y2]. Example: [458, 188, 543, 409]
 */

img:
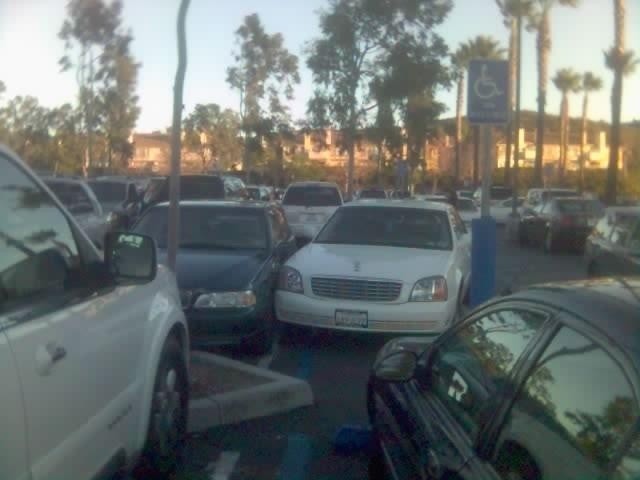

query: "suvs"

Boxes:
[1, 144, 193, 478]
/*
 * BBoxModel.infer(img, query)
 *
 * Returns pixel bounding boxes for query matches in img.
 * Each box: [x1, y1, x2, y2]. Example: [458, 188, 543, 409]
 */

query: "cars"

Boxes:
[134, 200, 297, 353]
[275, 199, 474, 343]
[366, 276, 640, 479]
